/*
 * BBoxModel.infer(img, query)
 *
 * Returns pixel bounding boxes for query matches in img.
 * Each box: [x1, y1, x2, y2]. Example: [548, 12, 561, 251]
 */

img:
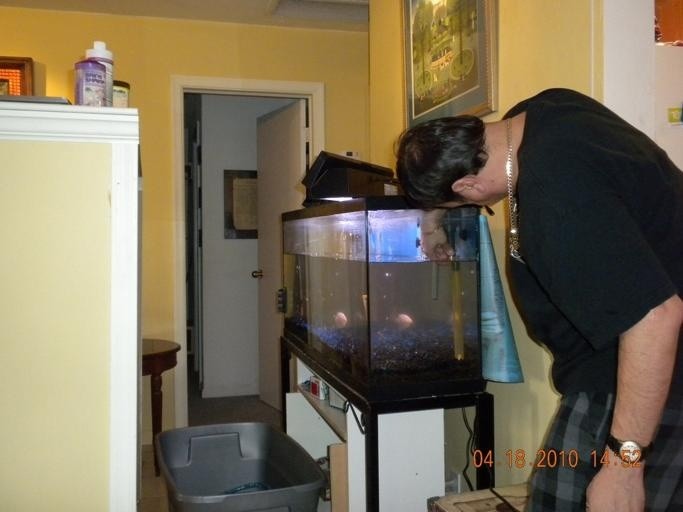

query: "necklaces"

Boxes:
[506, 117, 520, 259]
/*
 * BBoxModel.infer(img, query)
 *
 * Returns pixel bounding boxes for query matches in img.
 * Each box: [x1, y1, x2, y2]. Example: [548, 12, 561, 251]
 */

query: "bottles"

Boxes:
[73, 42, 130, 108]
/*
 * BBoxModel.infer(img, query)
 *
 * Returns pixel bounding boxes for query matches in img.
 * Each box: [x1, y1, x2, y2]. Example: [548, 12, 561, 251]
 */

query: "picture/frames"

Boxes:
[400, 0, 498, 133]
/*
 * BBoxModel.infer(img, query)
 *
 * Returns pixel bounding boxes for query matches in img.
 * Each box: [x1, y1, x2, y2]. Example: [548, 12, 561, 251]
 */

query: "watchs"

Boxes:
[605, 434, 655, 464]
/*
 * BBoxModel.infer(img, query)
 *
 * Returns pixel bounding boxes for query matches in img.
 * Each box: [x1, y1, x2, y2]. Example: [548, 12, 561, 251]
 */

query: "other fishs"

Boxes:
[395, 314, 413, 330]
[333, 312, 348, 328]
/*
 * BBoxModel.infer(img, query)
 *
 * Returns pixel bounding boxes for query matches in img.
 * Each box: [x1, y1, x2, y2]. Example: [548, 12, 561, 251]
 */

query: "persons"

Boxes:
[396, 88, 683, 512]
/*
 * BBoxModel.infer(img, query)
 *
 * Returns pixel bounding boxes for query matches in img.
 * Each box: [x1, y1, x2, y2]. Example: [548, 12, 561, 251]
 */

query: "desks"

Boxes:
[273, 335, 497, 512]
[140, 337, 181, 477]
